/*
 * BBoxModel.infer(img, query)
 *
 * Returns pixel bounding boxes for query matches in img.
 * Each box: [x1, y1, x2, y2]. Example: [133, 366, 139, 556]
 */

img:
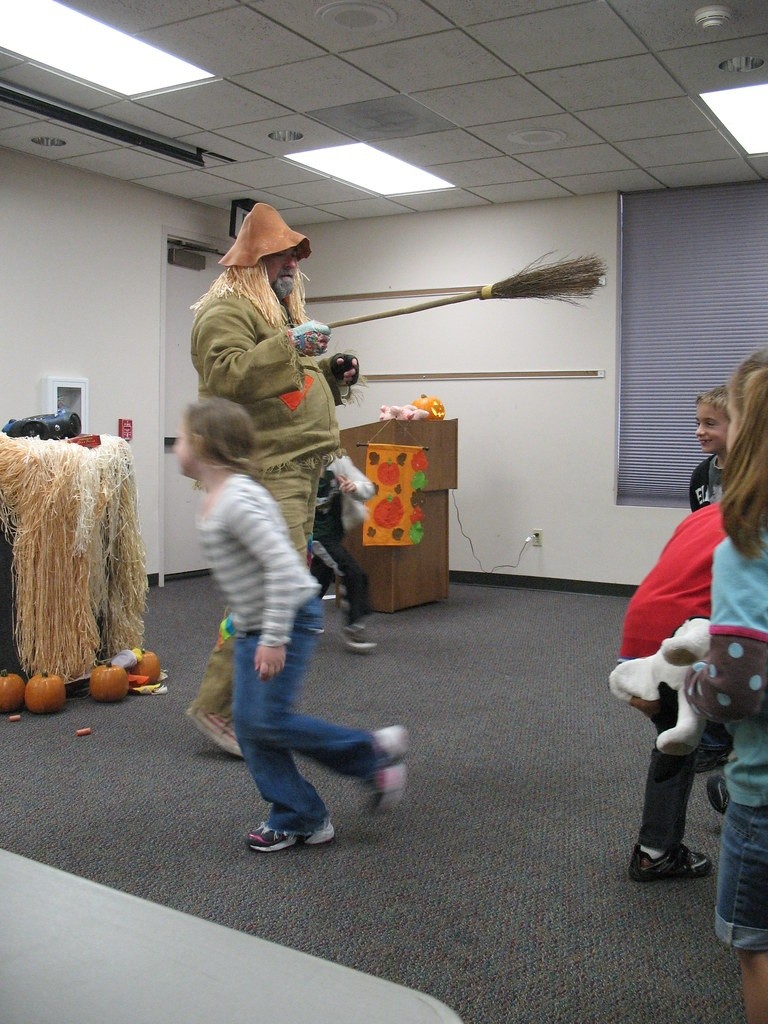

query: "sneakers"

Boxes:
[367, 724, 408, 814]
[339, 620, 380, 649]
[247, 814, 336, 852]
[705, 774, 730, 814]
[185, 703, 244, 759]
[627, 840, 713, 884]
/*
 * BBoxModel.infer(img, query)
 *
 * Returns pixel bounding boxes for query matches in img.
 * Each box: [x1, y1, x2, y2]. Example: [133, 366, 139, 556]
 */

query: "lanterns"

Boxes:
[411, 394, 446, 421]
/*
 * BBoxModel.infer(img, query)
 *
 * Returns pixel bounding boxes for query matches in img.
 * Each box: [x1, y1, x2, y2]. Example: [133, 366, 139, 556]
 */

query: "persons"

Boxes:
[173, 205, 359, 761]
[616, 348, 768, 1024]
[173, 395, 408, 852]
[310, 452, 377, 651]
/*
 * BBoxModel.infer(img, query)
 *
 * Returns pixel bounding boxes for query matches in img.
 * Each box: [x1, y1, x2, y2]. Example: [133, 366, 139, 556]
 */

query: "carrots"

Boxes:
[77, 727, 91, 735]
[9, 715, 20, 721]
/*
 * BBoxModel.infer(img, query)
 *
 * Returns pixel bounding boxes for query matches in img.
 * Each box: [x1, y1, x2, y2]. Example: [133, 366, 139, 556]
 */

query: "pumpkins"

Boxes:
[132, 649, 160, 685]
[0, 670, 26, 712]
[25, 671, 65, 713]
[90, 661, 129, 702]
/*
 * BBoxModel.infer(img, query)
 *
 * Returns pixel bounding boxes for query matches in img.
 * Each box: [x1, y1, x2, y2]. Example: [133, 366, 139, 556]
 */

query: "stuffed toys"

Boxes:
[609, 618, 711, 755]
[379, 405, 429, 420]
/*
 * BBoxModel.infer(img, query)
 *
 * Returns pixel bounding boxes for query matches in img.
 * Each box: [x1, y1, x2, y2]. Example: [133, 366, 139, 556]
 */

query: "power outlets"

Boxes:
[532, 529, 542, 546]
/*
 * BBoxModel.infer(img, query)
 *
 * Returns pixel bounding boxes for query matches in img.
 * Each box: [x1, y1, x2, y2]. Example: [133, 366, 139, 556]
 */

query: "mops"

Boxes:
[328, 250, 609, 330]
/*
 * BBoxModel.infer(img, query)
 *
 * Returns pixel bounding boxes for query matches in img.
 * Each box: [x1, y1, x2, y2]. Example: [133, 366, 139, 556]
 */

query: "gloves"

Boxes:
[291, 319, 332, 357]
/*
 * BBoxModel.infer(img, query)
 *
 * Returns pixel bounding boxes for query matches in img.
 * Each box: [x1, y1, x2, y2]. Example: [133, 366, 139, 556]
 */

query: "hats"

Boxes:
[217, 203, 312, 267]
